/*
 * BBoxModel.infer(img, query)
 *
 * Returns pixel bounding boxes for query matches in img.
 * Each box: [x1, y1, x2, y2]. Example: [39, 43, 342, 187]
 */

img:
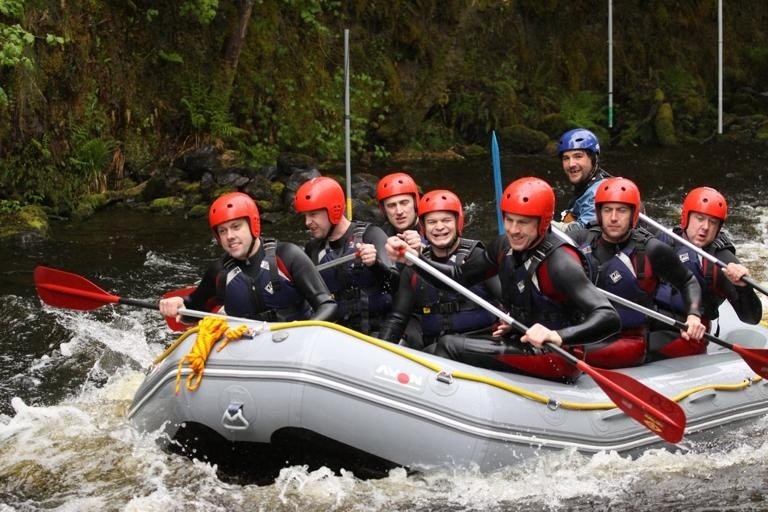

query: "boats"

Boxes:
[121, 300, 768, 488]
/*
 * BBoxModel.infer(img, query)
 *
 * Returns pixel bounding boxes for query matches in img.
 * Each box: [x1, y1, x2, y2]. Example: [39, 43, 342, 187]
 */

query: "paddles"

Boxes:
[395, 246, 687, 444]
[596, 287, 768, 381]
[34, 266, 265, 324]
[162, 243, 365, 331]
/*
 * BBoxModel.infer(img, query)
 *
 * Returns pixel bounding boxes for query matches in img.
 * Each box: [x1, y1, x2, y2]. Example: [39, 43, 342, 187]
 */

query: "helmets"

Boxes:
[294, 177, 346, 225]
[500, 176, 556, 237]
[376, 172, 420, 212]
[558, 130, 602, 156]
[680, 186, 729, 230]
[209, 191, 261, 247]
[417, 189, 464, 232]
[595, 177, 642, 228]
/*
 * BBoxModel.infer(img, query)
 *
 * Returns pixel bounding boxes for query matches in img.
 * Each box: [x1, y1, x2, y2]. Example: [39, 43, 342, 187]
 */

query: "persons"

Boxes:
[422, 176, 624, 385]
[376, 173, 426, 252]
[159, 192, 337, 324]
[573, 176, 705, 372]
[559, 126, 614, 249]
[374, 188, 505, 373]
[296, 177, 399, 352]
[638, 186, 764, 363]
